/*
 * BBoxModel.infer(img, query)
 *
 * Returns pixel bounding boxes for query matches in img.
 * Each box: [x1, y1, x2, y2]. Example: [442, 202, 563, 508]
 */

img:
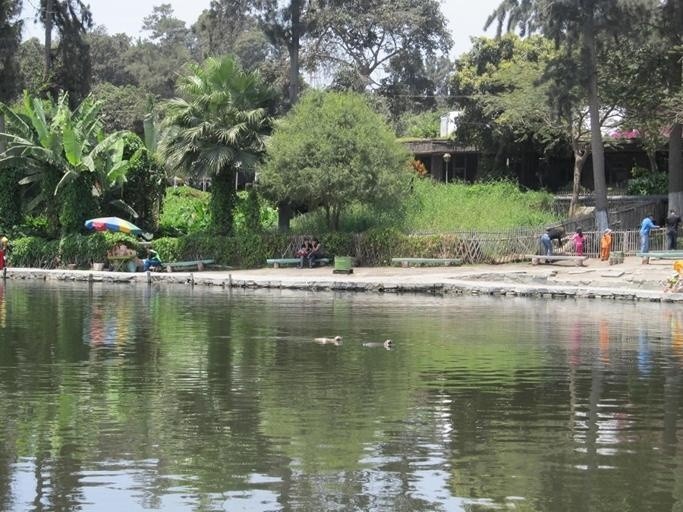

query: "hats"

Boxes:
[604, 228, 611, 236]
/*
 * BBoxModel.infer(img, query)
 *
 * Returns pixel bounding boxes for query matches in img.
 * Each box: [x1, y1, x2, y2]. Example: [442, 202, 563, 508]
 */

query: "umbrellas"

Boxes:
[84, 215, 142, 244]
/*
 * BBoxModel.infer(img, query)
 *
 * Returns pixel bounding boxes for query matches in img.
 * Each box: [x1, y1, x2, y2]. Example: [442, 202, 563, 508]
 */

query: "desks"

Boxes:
[107, 256, 134, 272]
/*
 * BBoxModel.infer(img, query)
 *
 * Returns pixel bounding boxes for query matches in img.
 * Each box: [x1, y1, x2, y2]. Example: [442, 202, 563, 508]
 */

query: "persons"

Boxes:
[664, 208, 681, 250]
[142, 247, 161, 271]
[571, 227, 588, 255]
[306, 237, 324, 269]
[598, 228, 613, 261]
[296, 237, 311, 269]
[541, 226, 565, 257]
[0, 236, 8, 270]
[106, 242, 137, 258]
[638, 215, 660, 253]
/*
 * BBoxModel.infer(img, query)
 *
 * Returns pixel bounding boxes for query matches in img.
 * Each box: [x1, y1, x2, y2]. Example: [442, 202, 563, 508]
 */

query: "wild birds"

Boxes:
[362, 339, 392, 347]
[314, 335, 344, 343]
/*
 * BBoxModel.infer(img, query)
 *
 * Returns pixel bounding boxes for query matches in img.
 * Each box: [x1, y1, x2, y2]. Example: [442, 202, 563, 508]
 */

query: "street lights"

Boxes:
[442, 152, 452, 185]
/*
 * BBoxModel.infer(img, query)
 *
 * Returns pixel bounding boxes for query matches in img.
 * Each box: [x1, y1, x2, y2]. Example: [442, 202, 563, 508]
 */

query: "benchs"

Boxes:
[266, 258, 330, 269]
[392, 257, 463, 268]
[161, 259, 215, 273]
[526, 251, 683, 266]
